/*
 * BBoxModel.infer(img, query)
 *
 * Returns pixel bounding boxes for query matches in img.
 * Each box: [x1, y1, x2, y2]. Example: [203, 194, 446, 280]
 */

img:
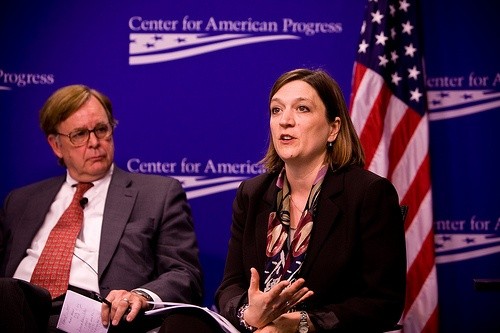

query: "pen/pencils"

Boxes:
[95, 293, 112, 306]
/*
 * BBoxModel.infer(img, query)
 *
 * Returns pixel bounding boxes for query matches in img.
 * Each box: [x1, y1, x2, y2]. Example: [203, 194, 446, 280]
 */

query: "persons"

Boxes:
[158, 69, 406, 333]
[0, 85, 201, 333]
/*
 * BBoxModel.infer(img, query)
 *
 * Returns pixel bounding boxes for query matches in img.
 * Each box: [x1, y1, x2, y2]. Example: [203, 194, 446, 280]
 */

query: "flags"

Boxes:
[349, 0, 439, 333]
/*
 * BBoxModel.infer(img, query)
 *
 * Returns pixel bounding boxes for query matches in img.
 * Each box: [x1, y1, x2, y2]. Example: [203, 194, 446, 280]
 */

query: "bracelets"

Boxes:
[236, 304, 257, 333]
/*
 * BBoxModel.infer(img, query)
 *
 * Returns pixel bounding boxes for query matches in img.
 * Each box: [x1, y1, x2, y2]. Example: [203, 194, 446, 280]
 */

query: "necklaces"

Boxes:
[290, 197, 303, 214]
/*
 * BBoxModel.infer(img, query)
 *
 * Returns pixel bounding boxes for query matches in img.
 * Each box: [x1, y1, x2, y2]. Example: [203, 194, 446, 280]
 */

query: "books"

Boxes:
[145, 301, 240, 333]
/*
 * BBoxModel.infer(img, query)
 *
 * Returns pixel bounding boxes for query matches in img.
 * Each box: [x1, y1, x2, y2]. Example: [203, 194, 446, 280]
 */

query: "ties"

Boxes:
[31, 182, 95, 300]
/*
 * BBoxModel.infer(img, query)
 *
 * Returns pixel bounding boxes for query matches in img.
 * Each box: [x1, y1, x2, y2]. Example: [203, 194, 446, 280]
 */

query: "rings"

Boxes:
[121, 297, 130, 305]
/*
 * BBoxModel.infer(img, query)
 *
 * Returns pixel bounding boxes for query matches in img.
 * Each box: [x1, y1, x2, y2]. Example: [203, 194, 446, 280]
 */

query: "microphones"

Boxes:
[79, 198, 88, 209]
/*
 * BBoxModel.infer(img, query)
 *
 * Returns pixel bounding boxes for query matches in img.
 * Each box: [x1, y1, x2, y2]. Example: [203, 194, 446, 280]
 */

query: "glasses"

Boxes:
[57, 122, 113, 145]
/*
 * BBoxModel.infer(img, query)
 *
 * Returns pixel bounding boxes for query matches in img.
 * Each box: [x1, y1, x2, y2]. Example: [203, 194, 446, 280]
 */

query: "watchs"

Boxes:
[297, 309, 309, 333]
[132, 290, 154, 309]
